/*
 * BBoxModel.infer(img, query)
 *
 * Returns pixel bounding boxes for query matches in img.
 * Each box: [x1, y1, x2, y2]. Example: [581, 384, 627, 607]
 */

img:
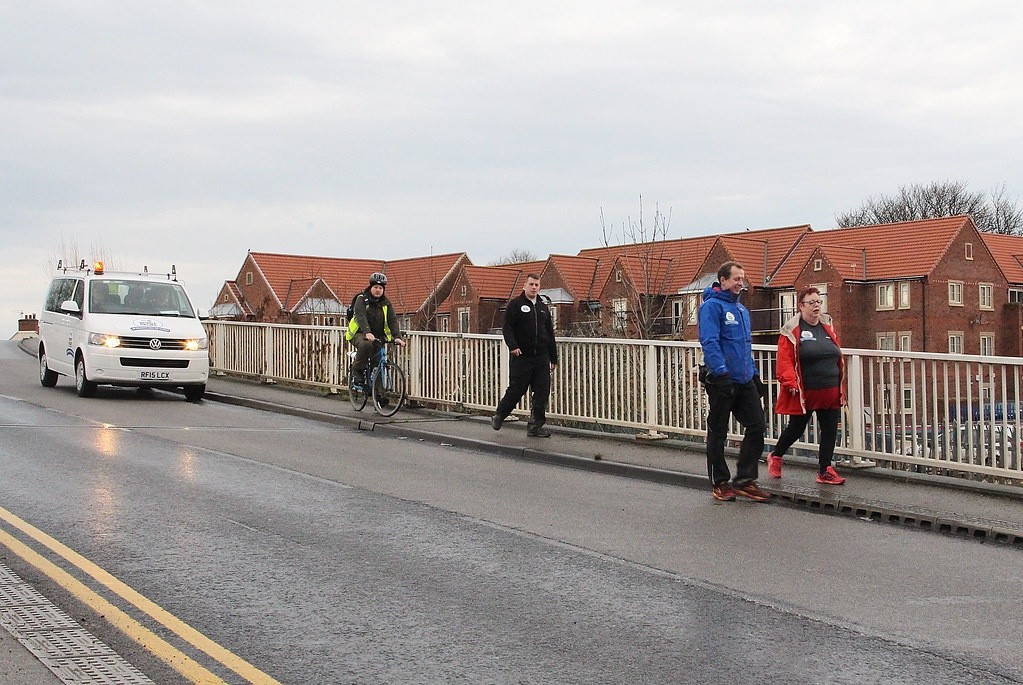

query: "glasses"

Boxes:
[802, 299, 823, 306]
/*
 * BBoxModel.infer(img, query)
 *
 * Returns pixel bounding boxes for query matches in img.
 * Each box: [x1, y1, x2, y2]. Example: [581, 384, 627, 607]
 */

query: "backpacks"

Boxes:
[346, 292, 371, 323]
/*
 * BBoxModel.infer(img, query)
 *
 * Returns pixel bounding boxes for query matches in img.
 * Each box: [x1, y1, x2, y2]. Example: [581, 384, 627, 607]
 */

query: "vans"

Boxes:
[38, 259, 210, 402]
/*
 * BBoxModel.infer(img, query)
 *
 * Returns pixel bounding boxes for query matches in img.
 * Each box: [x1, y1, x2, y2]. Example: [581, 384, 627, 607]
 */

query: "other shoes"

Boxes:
[375, 397, 390, 411]
[527, 427, 551, 438]
[352, 383, 364, 392]
[492, 415, 504, 431]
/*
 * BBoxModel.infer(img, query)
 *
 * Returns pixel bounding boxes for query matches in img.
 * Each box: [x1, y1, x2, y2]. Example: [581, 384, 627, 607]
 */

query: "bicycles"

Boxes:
[346, 337, 407, 418]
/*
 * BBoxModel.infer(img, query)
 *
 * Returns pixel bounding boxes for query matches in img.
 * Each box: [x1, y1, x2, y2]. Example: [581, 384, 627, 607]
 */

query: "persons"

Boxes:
[346, 273, 406, 410]
[491, 272, 557, 437]
[149, 288, 178, 311]
[91, 283, 112, 306]
[697, 261, 771, 501]
[767, 288, 845, 484]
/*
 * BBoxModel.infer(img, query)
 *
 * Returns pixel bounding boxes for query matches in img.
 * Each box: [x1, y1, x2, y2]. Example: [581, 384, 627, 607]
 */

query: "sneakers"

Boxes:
[711, 483, 736, 501]
[732, 480, 771, 501]
[816, 466, 846, 485]
[766, 451, 783, 479]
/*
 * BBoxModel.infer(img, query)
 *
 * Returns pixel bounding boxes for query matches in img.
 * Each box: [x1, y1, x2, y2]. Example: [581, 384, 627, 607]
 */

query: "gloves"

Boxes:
[715, 372, 736, 412]
[752, 375, 766, 398]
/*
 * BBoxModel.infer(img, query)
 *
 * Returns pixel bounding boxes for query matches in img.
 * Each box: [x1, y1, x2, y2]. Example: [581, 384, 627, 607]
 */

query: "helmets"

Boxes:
[369, 273, 388, 286]
